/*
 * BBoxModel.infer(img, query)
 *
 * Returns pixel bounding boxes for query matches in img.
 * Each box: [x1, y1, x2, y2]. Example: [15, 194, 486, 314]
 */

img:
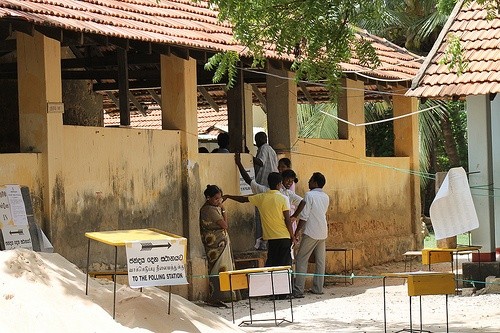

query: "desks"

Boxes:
[218, 265, 294, 327]
[84, 227, 190, 319]
[324, 248, 354, 286]
[421, 245, 483, 295]
[402, 251, 422, 287]
[380, 271, 457, 333]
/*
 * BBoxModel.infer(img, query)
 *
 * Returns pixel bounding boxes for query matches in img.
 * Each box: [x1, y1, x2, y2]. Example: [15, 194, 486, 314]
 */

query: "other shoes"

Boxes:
[256, 244, 267, 250]
[304, 289, 322, 294]
[285, 292, 304, 299]
[207, 298, 228, 308]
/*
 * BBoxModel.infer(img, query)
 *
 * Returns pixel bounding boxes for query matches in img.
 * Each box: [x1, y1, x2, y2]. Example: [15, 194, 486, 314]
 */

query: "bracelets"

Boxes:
[221, 211, 225, 214]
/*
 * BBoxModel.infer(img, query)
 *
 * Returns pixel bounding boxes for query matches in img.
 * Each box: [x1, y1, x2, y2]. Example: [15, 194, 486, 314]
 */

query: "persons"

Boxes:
[199, 184, 242, 308]
[198, 132, 330, 300]
